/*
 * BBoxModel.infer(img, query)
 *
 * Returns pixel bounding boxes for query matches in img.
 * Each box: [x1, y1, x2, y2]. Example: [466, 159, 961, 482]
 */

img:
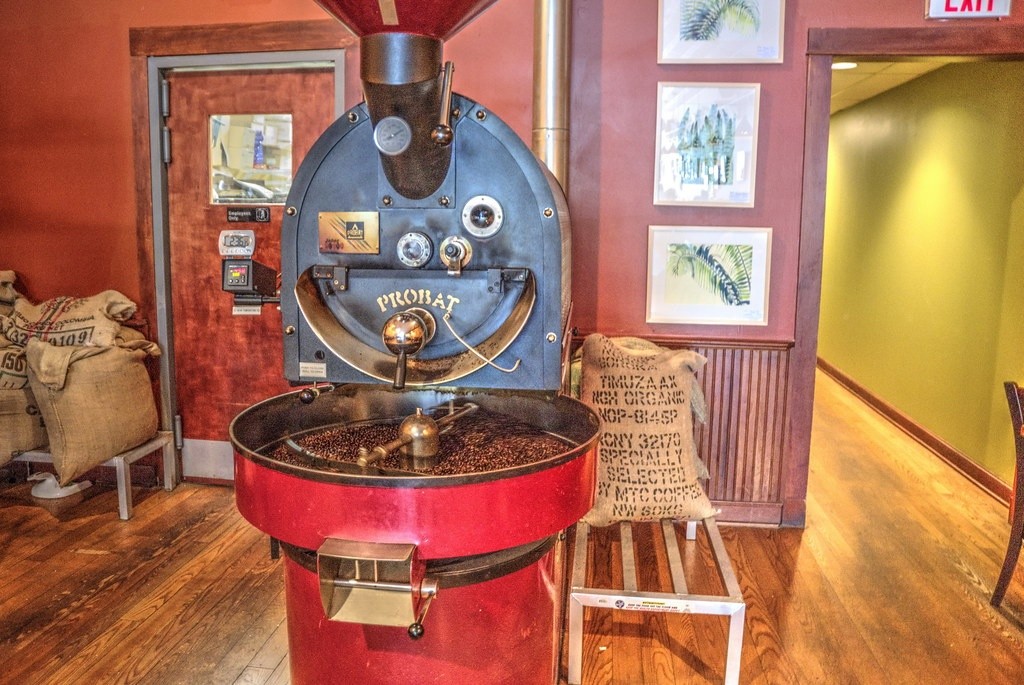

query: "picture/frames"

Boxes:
[653, 82, 761, 208]
[657, 0, 786, 64]
[645, 225, 773, 326]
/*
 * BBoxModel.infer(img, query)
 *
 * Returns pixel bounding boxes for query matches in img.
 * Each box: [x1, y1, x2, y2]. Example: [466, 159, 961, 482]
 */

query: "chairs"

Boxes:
[990, 381, 1024, 609]
